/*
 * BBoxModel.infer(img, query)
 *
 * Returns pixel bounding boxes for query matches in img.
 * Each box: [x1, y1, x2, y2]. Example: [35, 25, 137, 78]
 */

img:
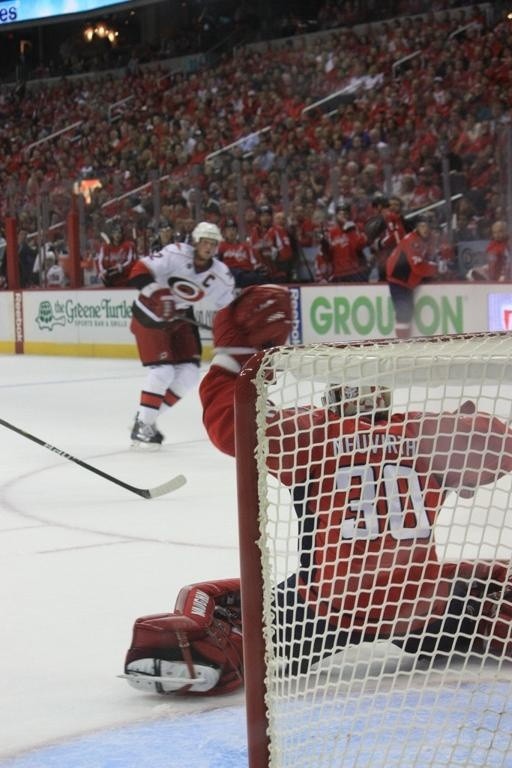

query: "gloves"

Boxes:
[151, 288, 175, 319]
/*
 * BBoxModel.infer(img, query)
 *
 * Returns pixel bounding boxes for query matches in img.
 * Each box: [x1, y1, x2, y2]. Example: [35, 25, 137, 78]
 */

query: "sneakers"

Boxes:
[132, 411, 162, 443]
[126, 658, 222, 694]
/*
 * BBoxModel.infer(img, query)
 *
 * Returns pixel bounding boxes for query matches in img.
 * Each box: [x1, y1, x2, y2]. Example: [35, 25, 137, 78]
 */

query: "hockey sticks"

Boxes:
[1, 420, 187, 499]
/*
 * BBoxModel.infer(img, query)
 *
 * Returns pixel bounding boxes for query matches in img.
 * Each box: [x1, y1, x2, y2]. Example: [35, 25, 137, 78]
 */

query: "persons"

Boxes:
[123, 299, 512, 697]
[125, 221, 239, 445]
[1, 1, 512, 290]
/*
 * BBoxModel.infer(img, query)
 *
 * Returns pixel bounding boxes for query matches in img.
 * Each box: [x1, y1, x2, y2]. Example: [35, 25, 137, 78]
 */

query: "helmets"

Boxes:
[192, 222, 223, 243]
[335, 204, 350, 224]
[321, 384, 392, 416]
[259, 206, 272, 226]
